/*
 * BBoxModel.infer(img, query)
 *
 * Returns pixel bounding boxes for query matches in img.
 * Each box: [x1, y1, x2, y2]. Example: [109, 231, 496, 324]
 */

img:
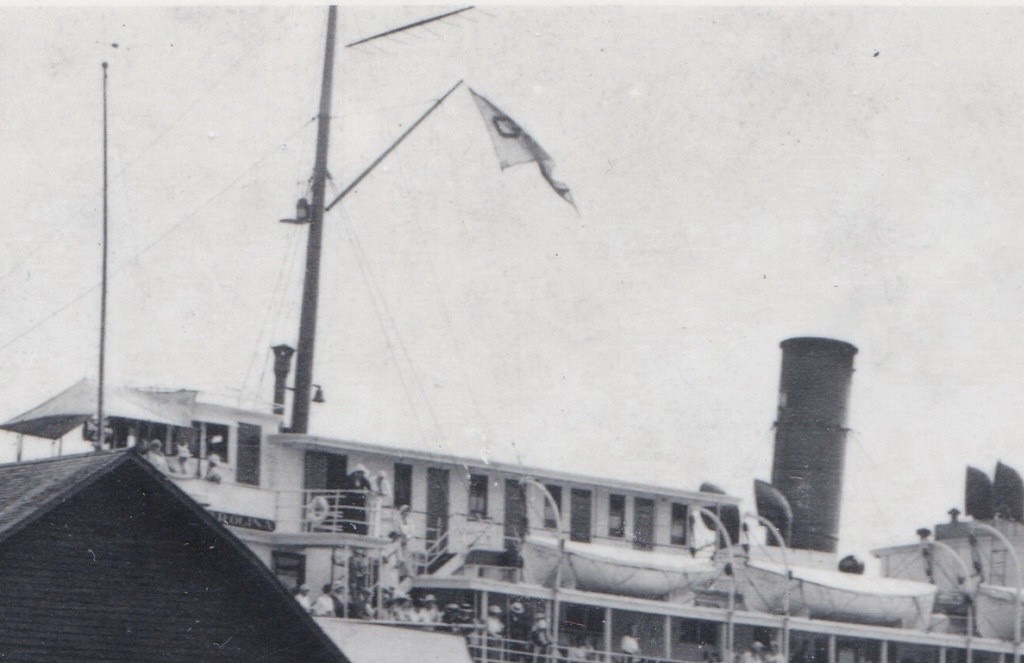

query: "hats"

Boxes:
[749, 640, 779, 649]
[298, 580, 545, 621]
[175, 444, 193, 458]
[208, 454, 220, 466]
[352, 463, 388, 479]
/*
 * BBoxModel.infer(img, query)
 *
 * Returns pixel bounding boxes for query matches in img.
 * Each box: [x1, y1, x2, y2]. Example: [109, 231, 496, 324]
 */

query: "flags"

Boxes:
[471, 86, 578, 219]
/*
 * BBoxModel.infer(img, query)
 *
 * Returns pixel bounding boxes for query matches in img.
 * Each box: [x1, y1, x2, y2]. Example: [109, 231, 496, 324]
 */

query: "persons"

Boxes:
[388, 503, 416, 569]
[290, 580, 641, 663]
[136, 437, 223, 486]
[698, 633, 830, 663]
[364, 470, 394, 538]
[341, 464, 375, 535]
